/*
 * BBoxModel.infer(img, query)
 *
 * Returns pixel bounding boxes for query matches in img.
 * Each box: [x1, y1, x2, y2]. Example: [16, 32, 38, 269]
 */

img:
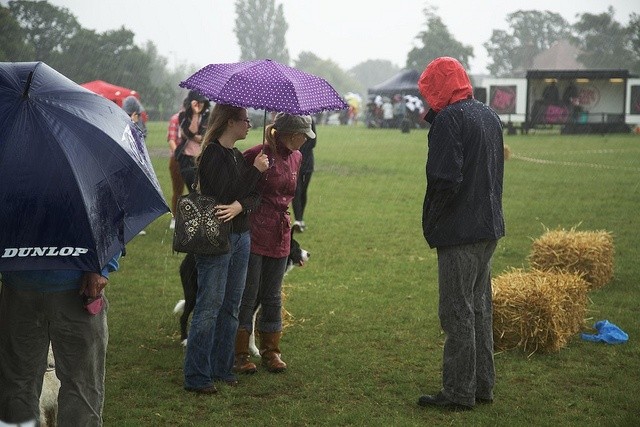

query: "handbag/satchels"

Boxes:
[173, 195, 231, 256]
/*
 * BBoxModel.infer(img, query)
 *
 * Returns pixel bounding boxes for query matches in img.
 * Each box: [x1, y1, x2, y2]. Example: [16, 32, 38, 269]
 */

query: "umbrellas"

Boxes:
[405, 96, 424, 113]
[178, 59, 349, 154]
[80, 80, 139, 107]
[1, 61, 172, 276]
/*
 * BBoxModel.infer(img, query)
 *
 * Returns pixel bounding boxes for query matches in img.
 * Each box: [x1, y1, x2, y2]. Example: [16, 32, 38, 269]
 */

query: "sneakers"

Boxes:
[184, 382, 218, 393]
[216, 373, 239, 385]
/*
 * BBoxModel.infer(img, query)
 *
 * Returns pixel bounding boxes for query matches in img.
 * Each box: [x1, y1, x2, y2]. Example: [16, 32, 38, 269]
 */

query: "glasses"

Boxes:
[237, 118, 250, 124]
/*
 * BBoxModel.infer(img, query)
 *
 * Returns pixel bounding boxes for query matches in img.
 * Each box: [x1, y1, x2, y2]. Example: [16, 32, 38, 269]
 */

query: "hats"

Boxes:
[274, 112, 316, 139]
[189, 90, 209, 103]
[122, 95, 144, 114]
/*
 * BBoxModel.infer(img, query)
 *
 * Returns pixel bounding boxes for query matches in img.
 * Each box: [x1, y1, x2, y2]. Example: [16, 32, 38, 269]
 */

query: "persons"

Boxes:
[234, 114, 315, 371]
[292, 118, 317, 232]
[367, 93, 404, 127]
[417, 57, 506, 410]
[339, 104, 349, 125]
[1, 252, 123, 427]
[122, 96, 147, 138]
[168, 92, 212, 231]
[184, 102, 269, 392]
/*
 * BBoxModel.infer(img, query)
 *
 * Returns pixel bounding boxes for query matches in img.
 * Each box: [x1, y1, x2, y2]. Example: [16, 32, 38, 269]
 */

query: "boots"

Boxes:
[259, 332, 287, 372]
[231, 328, 258, 374]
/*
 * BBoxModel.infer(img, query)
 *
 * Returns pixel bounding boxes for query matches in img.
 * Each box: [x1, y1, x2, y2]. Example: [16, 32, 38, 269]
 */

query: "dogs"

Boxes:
[172, 219, 311, 359]
[39, 341, 62, 427]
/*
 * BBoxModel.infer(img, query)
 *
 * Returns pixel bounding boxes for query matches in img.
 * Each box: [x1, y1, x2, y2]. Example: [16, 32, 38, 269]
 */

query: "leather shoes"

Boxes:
[416, 391, 472, 411]
[476, 394, 493, 405]
